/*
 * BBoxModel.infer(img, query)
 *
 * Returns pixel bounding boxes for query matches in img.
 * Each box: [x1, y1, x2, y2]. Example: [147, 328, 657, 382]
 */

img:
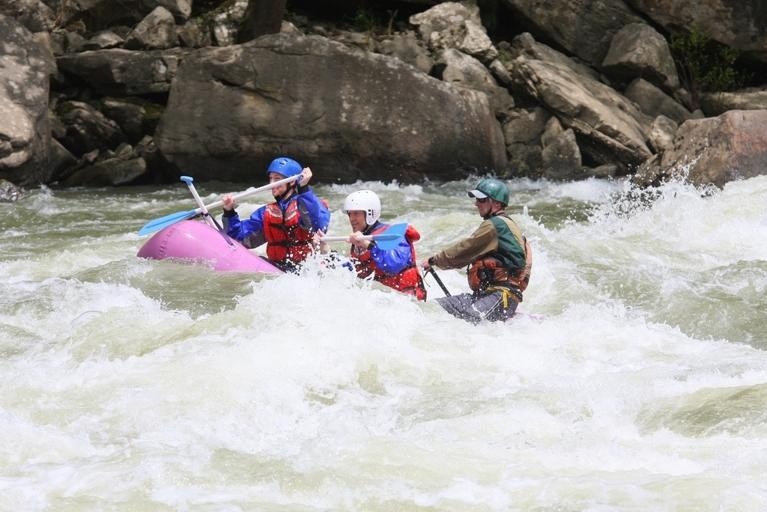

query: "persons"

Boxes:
[221, 156, 331, 275]
[312, 188, 425, 302]
[418, 176, 531, 325]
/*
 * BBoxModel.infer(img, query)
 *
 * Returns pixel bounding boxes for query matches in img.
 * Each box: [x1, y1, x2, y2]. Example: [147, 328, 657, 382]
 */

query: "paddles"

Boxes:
[137, 167, 303, 237]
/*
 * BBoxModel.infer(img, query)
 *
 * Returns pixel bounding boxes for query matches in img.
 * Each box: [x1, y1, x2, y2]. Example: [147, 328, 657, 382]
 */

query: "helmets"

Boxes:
[468, 178, 509, 207]
[343, 190, 381, 226]
[266, 157, 302, 184]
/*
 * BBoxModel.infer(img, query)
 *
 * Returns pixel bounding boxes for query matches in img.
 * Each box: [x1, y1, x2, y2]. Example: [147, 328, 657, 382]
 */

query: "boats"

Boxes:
[135, 219, 286, 278]
